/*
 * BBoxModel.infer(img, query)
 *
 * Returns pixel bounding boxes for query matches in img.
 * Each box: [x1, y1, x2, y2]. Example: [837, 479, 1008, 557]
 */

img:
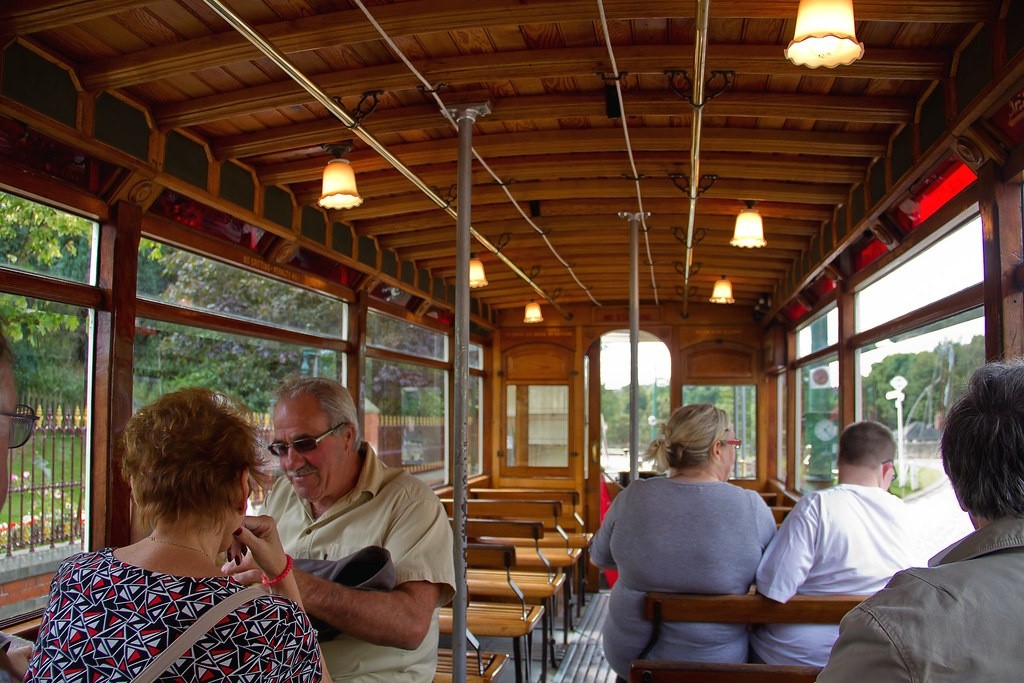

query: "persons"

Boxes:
[584, 401, 788, 683]
[221, 375, 460, 683]
[18, 386, 333, 681]
[0, 331, 47, 683]
[810, 356, 1024, 683]
[751, 420, 935, 675]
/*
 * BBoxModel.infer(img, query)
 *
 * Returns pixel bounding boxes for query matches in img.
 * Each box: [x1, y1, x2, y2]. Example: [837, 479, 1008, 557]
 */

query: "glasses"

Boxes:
[719, 438, 743, 450]
[267, 422, 345, 457]
[882, 459, 898, 481]
[0, 404, 35, 449]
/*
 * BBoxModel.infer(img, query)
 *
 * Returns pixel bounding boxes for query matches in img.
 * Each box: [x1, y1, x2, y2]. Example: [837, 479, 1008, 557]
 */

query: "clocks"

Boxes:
[813, 419, 836, 442]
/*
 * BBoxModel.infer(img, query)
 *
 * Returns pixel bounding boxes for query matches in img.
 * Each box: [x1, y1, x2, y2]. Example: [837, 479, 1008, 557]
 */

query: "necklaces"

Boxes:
[141, 534, 211, 559]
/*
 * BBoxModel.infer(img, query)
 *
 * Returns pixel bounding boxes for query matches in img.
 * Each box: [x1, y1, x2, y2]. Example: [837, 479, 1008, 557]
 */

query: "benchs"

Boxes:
[409, 481, 879, 683]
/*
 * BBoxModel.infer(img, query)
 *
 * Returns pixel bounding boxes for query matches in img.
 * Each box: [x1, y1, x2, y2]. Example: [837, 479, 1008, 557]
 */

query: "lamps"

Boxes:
[728, 199, 768, 250]
[468, 252, 490, 289]
[317, 139, 364, 210]
[783, 0, 865, 70]
[708, 276, 735, 304]
[522, 299, 543, 324]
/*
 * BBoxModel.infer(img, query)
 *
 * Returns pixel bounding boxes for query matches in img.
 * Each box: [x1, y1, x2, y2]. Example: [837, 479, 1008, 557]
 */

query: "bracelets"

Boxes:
[258, 554, 294, 586]
